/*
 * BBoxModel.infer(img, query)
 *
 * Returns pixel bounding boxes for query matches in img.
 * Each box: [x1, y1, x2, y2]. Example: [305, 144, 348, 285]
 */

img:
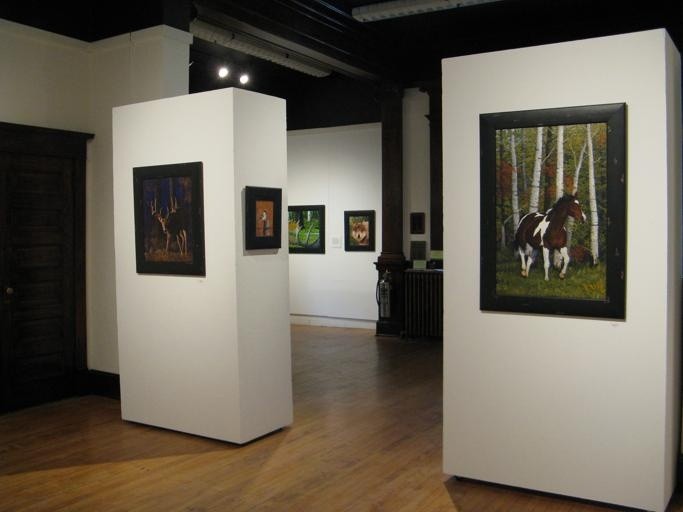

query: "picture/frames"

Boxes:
[288, 205, 376, 254]
[132, 161, 284, 278]
[477, 101, 627, 321]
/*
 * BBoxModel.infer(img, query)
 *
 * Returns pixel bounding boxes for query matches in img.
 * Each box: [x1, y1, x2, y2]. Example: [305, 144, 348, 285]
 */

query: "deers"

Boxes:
[150, 196, 189, 257]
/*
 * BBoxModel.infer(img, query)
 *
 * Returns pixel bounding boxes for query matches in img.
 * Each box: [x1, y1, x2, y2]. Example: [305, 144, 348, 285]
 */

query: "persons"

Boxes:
[257, 210, 270, 237]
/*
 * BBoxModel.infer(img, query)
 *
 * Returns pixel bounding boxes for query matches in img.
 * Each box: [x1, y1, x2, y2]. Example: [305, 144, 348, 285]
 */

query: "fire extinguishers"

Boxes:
[376, 272, 392, 319]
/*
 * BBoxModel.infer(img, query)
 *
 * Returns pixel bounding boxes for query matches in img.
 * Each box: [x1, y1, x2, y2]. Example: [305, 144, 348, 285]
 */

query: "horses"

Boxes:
[516, 191, 587, 281]
